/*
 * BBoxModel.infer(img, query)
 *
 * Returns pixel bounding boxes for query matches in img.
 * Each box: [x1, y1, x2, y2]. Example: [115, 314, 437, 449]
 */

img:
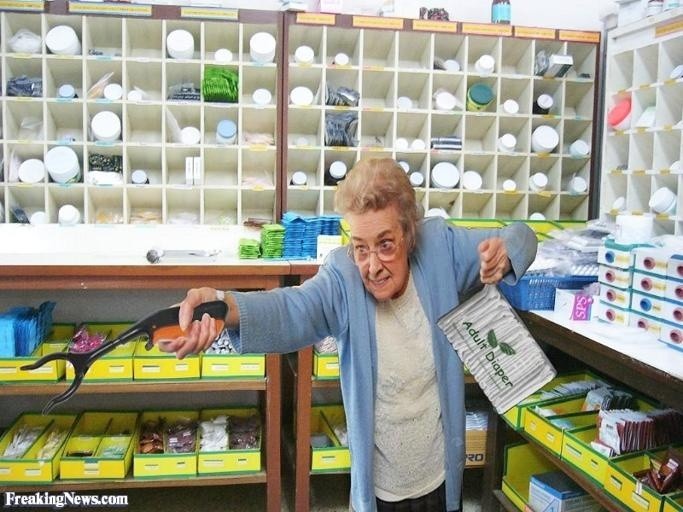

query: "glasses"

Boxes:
[345, 233, 406, 268]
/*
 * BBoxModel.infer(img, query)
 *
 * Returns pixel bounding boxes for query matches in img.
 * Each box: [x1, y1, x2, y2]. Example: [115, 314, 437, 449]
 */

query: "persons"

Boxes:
[156, 157, 538, 510]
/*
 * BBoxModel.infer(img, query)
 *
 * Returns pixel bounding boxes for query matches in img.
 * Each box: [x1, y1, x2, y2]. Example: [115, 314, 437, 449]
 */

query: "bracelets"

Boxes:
[215, 289, 224, 300]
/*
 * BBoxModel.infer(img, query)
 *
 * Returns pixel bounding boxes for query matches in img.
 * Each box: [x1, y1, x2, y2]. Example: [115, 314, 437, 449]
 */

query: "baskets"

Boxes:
[497, 274, 598, 312]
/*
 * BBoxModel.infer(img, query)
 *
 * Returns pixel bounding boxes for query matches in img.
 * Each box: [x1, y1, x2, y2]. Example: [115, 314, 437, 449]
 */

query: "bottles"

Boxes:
[646, 0, 663, 18]
[606, 97, 632, 131]
[648, 186, 677, 218]
[613, 196, 627, 212]
[668, 64, 682, 79]
[489, 1, 513, 27]
[18, 22, 590, 223]
[662, 1, 680, 12]
